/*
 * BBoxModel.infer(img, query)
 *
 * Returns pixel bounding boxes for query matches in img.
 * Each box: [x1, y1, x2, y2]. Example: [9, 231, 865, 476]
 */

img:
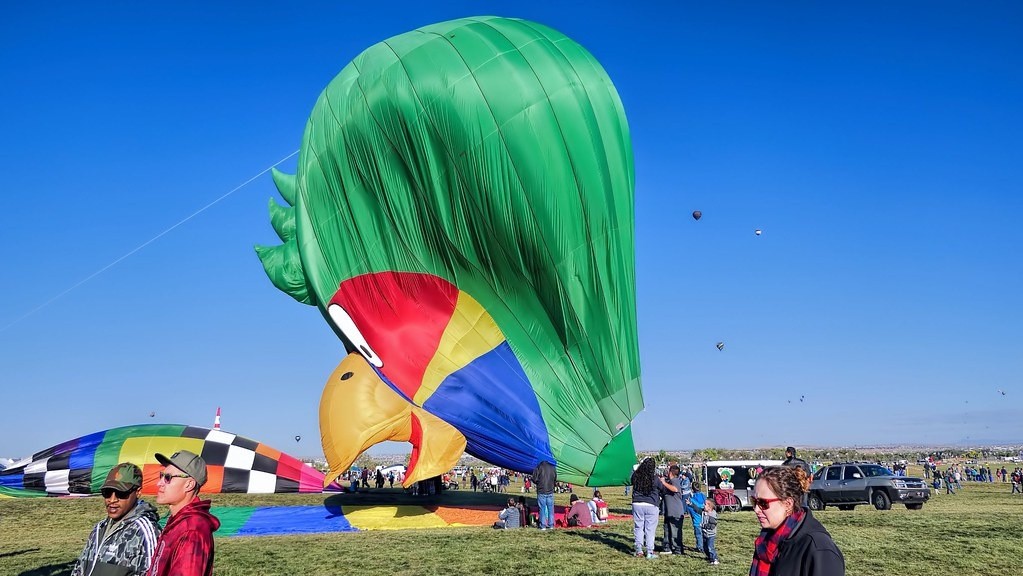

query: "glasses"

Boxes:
[159, 471, 190, 484]
[748, 495, 782, 510]
[101, 488, 136, 499]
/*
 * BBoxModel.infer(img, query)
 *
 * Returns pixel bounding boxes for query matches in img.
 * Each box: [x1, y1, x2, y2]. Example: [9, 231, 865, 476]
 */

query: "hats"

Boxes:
[570, 494, 578, 505]
[101, 463, 143, 492]
[154, 450, 207, 486]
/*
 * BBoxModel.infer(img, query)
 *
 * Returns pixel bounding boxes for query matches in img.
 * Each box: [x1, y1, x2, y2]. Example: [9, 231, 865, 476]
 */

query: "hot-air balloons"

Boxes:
[717, 341, 724, 352]
[1, 406, 351, 499]
[787, 394, 804, 404]
[255, 11, 646, 489]
[692, 210, 702, 221]
[1002, 391, 1006, 396]
[755, 229, 762, 236]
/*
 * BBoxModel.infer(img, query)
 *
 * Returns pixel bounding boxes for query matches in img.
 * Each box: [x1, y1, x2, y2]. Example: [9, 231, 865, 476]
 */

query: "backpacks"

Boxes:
[948, 477, 953, 482]
[593, 500, 609, 520]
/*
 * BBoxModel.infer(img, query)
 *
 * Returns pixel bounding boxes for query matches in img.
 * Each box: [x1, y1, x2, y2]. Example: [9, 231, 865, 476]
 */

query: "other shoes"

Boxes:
[697, 548, 703, 553]
[646, 550, 659, 559]
[711, 559, 720, 565]
[1019, 491, 1022, 495]
[541, 527, 547, 531]
[636, 551, 644, 557]
[660, 548, 672, 555]
[1012, 493, 1016, 495]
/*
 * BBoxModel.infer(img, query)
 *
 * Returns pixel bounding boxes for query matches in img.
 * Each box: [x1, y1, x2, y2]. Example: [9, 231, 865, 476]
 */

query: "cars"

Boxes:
[806, 462, 931, 511]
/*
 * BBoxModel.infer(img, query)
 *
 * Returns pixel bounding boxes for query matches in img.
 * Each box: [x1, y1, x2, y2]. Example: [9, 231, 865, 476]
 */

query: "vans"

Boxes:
[701, 458, 785, 513]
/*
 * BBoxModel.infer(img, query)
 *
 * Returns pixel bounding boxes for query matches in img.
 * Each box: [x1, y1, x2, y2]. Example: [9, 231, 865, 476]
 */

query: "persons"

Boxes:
[686, 498, 719, 564]
[625, 486, 630, 495]
[71, 463, 162, 576]
[631, 457, 667, 559]
[782, 447, 813, 506]
[493, 498, 520, 529]
[687, 481, 707, 552]
[749, 466, 845, 576]
[658, 465, 684, 555]
[147, 450, 220, 576]
[587, 491, 607, 523]
[515, 496, 530, 527]
[531, 461, 556, 531]
[442, 466, 531, 493]
[566, 494, 592, 526]
[339, 467, 401, 491]
[888, 462, 907, 476]
[655, 464, 698, 516]
[916, 451, 1023, 496]
[555, 481, 572, 493]
